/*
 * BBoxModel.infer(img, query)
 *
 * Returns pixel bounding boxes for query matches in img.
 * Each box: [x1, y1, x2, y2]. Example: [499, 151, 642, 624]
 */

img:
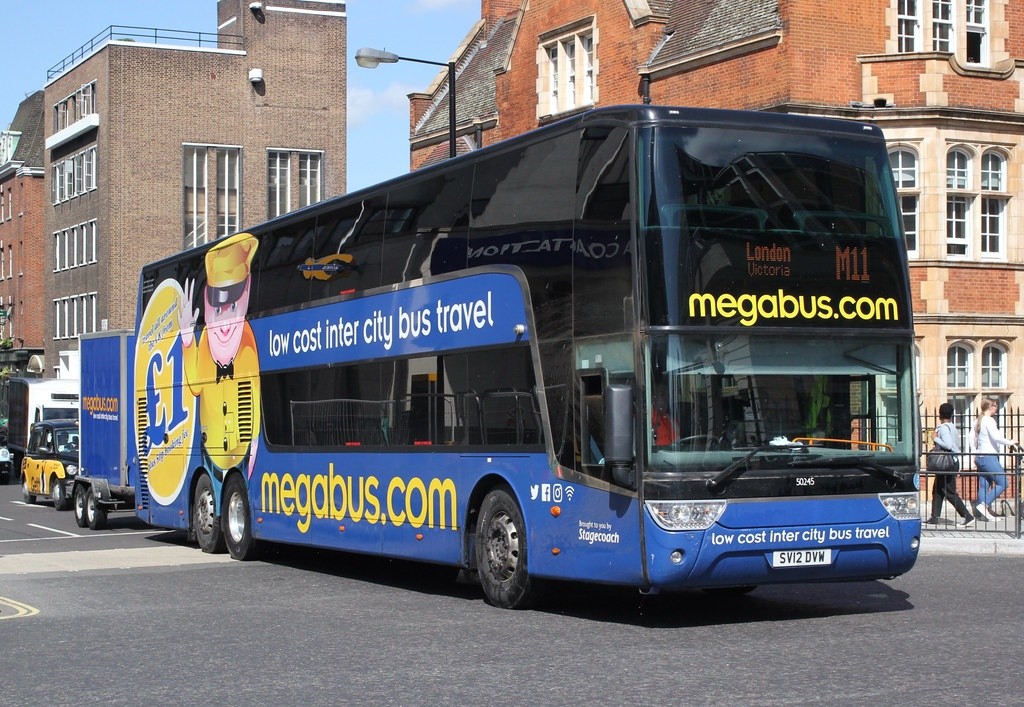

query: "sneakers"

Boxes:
[976, 504, 993, 519]
[980, 514, 1001, 522]
[958, 514, 975, 528]
[921, 517, 938, 526]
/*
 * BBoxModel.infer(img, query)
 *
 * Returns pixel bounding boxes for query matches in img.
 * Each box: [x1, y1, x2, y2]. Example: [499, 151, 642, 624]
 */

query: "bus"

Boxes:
[128, 104, 925, 613]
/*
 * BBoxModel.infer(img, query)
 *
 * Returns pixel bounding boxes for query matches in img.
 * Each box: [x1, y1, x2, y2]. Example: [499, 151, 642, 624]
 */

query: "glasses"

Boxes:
[992, 405, 998, 408]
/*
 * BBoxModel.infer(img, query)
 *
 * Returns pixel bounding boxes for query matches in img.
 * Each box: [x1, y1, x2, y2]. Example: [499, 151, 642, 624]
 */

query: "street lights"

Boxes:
[354, 47, 456, 158]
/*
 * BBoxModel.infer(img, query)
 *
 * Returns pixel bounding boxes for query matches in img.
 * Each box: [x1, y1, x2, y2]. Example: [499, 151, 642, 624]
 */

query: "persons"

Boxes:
[921, 403, 975, 528]
[59, 432, 69, 444]
[968, 398, 1019, 521]
[652, 396, 680, 446]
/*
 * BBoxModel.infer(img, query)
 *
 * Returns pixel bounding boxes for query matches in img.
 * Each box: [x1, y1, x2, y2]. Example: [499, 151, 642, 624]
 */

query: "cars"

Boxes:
[20, 418, 80, 510]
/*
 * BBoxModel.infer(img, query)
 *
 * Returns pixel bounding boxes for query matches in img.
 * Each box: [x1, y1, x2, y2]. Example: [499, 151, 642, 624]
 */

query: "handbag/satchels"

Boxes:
[926, 424, 955, 471]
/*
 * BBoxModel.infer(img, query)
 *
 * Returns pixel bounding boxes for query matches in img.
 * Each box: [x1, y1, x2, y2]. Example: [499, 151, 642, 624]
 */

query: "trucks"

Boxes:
[7, 378, 79, 476]
[69, 327, 139, 531]
